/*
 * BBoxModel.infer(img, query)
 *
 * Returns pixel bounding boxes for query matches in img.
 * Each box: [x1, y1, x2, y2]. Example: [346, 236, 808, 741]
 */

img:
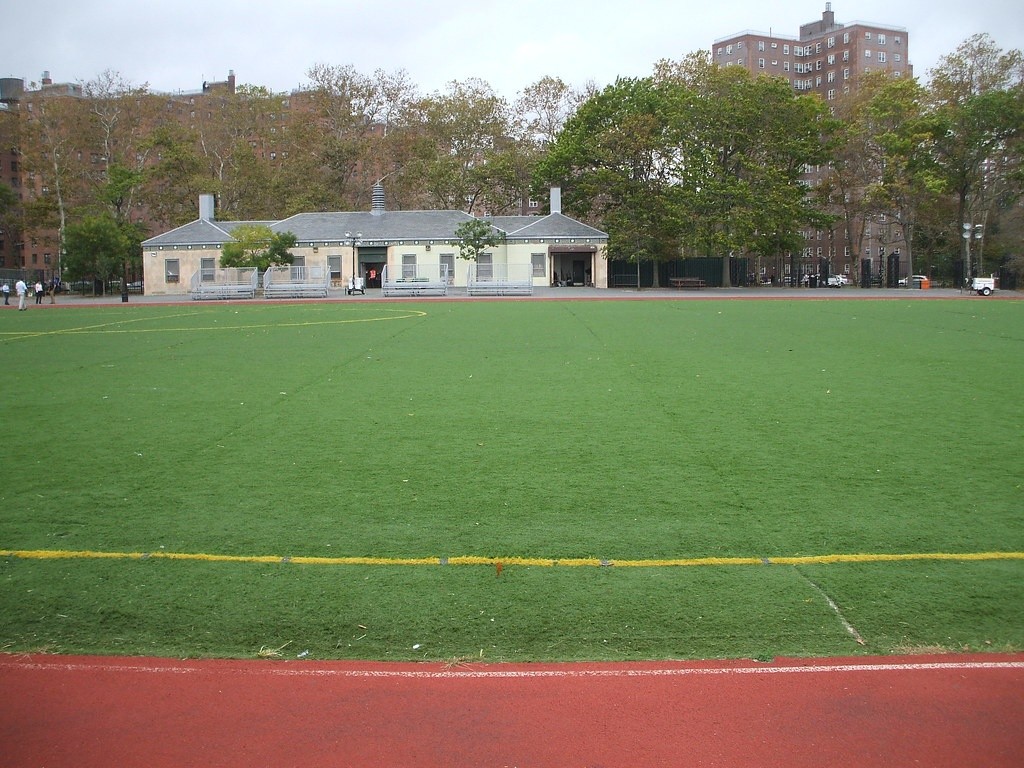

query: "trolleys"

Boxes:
[971, 276, 995, 297]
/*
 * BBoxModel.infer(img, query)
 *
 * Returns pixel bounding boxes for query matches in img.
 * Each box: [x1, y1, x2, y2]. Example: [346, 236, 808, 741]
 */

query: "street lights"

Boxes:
[344, 231, 364, 295]
[962, 222, 983, 291]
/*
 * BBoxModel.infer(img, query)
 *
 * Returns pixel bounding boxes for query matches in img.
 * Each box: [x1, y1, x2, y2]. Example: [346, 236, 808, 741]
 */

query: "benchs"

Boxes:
[192, 284, 328, 300]
[383, 277, 532, 296]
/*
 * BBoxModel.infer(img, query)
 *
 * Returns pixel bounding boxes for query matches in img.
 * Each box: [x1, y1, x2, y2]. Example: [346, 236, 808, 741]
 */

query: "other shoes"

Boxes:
[24, 308, 27, 311]
[19, 309, 22, 311]
[36, 302, 38, 304]
[374, 286, 376, 288]
[39, 302, 41, 304]
[5, 303, 9, 305]
[370, 286, 373, 288]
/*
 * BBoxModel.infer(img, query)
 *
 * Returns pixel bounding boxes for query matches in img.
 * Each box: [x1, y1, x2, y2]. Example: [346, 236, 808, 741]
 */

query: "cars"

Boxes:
[778, 273, 853, 288]
[899, 275, 927, 286]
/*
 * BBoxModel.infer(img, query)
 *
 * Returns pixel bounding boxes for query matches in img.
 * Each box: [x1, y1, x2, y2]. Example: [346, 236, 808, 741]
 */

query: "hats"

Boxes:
[370, 267, 375, 270]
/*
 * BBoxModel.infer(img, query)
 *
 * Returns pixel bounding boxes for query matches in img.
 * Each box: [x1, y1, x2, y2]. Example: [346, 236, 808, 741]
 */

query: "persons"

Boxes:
[16, 277, 28, 310]
[554, 271, 557, 287]
[48, 278, 61, 304]
[35, 280, 43, 304]
[567, 271, 572, 279]
[803, 274, 809, 287]
[3, 283, 10, 305]
[770, 275, 775, 287]
[819, 277, 844, 288]
[366, 268, 377, 288]
[586, 266, 591, 285]
